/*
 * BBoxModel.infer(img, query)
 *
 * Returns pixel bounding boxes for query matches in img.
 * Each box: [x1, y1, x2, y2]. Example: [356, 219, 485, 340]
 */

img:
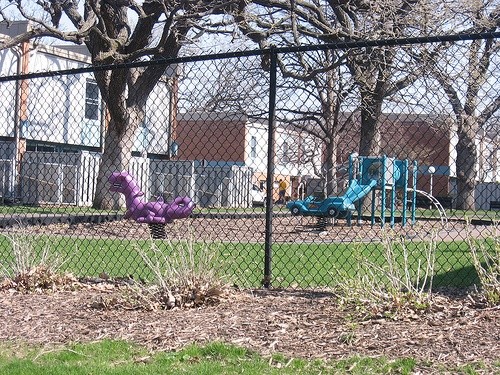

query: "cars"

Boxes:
[252, 184, 268, 208]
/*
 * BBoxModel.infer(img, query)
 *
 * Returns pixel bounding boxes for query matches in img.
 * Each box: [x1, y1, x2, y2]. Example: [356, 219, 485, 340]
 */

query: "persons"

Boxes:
[314, 187, 325, 201]
[278, 179, 287, 204]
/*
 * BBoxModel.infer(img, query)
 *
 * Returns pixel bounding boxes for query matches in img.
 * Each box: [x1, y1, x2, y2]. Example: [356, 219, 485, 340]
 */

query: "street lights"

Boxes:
[427, 166, 436, 211]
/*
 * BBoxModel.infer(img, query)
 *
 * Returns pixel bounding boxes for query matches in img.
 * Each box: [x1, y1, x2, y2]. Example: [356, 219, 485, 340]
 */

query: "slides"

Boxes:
[343, 179, 377, 205]
